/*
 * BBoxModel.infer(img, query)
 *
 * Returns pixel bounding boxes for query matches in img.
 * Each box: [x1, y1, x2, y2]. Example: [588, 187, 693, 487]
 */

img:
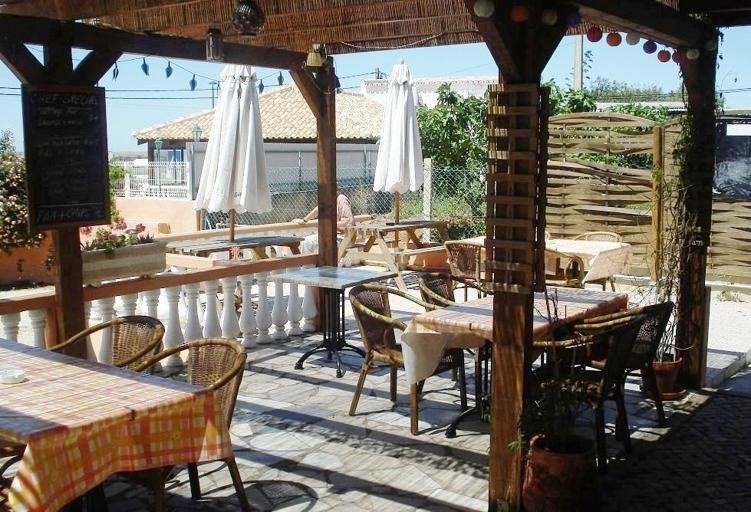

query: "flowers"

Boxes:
[79, 215, 158, 261]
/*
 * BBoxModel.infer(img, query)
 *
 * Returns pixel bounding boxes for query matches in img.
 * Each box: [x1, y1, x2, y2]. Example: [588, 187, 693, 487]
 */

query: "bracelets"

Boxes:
[304, 218, 308, 222]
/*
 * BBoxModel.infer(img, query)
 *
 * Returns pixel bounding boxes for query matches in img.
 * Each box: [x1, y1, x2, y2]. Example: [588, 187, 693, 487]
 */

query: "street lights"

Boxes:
[154, 138, 164, 198]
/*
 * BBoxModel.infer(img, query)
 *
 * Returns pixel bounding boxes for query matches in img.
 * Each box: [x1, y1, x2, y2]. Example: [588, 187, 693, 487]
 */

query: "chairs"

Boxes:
[541, 241, 588, 289]
[349, 283, 469, 439]
[46, 313, 167, 375]
[115, 336, 252, 510]
[585, 298, 673, 441]
[446, 240, 492, 299]
[531, 314, 648, 468]
[417, 270, 493, 382]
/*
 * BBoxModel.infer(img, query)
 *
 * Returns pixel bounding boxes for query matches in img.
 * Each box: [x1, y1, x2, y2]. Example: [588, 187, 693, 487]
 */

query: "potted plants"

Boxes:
[479, 286, 604, 512]
[609, 76, 730, 401]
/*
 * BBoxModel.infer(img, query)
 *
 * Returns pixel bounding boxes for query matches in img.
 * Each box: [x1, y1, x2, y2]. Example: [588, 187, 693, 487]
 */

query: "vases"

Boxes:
[80, 240, 172, 290]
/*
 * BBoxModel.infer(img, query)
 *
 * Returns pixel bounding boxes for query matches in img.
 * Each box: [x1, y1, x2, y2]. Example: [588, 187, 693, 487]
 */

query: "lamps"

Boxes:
[203, 27, 227, 66]
[231, 1, 268, 37]
[305, 39, 330, 68]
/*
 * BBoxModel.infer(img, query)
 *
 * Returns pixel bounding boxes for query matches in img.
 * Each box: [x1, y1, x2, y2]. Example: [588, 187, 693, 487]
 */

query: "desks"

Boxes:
[0, 335, 236, 511]
[338, 219, 452, 296]
[166, 235, 307, 258]
[408, 290, 631, 444]
[265, 264, 400, 379]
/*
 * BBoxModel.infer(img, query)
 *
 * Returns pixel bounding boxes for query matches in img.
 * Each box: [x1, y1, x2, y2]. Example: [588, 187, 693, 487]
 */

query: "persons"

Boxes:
[288, 188, 356, 268]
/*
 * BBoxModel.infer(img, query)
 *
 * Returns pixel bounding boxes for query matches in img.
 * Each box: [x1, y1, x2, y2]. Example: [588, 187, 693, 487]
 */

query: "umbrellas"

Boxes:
[194, 63, 274, 243]
[372, 56, 425, 248]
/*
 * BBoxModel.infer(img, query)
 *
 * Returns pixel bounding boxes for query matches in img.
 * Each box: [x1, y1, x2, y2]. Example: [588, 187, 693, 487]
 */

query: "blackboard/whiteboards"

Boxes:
[21, 83, 112, 234]
